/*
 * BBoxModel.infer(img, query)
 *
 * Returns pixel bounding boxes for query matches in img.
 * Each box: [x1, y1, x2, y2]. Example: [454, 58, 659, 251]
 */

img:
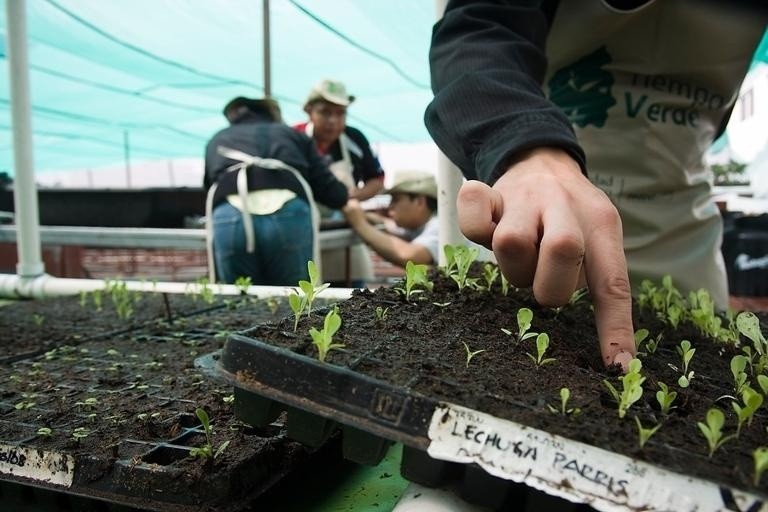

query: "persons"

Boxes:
[424, 0, 768, 376]
[201, 92, 348, 286]
[343, 172, 442, 277]
[291, 81, 386, 281]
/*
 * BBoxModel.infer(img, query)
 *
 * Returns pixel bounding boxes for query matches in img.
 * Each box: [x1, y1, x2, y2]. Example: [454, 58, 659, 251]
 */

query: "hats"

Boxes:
[382, 175, 437, 198]
[304, 80, 354, 113]
[224, 97, 281, 123]
